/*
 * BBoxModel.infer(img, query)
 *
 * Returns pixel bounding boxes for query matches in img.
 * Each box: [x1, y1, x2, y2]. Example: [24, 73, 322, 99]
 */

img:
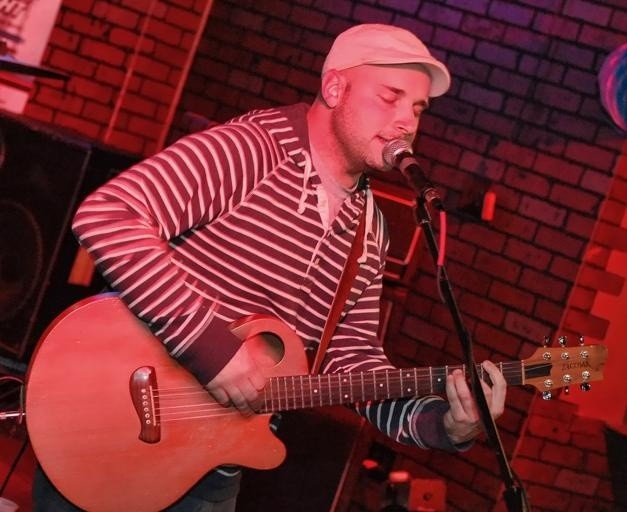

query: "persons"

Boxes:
[32, 23, 509, 511]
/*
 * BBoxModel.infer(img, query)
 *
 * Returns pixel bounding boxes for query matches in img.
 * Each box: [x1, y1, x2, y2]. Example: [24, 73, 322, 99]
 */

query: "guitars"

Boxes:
[24, 292, 608, 512]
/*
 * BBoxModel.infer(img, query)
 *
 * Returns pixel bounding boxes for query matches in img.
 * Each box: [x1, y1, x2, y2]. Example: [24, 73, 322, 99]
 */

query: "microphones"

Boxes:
[382, 139, 444, 211]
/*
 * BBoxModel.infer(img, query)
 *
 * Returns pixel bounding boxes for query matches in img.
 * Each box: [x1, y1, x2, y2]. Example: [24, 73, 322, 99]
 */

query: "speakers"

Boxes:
[0, 109, 147, 380]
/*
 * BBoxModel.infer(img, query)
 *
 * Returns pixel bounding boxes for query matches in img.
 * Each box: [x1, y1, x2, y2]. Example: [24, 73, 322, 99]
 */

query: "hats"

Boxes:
[322, 25, 451, 98]
